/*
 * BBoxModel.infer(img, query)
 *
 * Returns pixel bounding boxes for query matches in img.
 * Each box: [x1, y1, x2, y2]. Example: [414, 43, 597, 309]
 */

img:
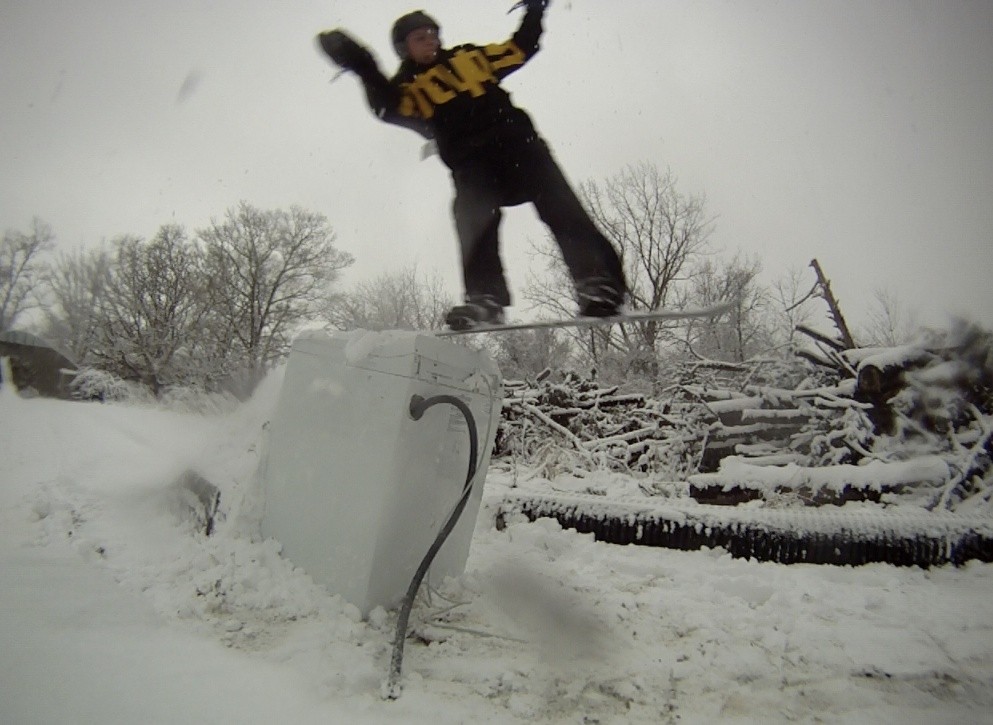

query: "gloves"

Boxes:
[319, 30, 378, 80]
[523, 0, 543, 12]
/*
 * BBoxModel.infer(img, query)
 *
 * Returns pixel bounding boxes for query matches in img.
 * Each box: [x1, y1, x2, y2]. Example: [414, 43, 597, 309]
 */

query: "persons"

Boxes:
[317, 0, 626, 333]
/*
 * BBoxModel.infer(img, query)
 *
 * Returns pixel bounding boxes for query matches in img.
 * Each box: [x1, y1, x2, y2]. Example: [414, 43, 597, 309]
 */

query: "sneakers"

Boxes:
[446, 299, 505, 329]
[577, 284, 623, 317]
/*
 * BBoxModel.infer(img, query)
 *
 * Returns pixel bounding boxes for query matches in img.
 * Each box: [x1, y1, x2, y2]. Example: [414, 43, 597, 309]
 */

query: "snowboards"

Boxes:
[434, 302, 736, 336]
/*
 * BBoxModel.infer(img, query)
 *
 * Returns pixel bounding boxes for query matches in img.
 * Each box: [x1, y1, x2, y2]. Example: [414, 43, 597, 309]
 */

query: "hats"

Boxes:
[392, 12, 439, 42]
